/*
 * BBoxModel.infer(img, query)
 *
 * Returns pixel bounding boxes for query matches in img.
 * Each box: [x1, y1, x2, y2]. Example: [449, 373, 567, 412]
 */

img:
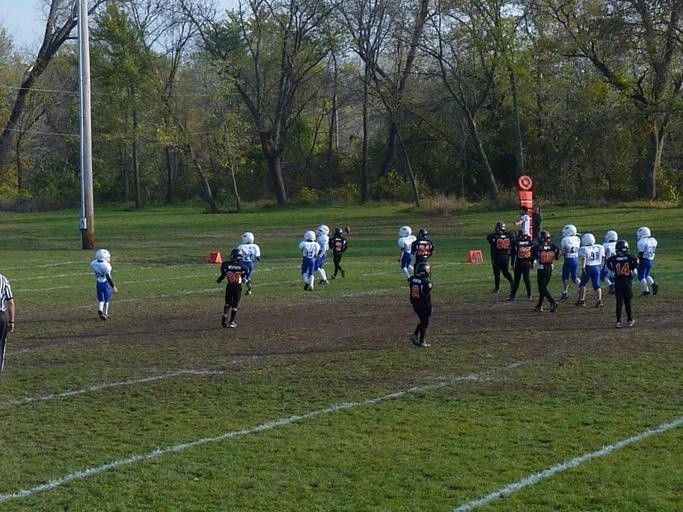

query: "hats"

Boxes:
[519, 205, 528, 212]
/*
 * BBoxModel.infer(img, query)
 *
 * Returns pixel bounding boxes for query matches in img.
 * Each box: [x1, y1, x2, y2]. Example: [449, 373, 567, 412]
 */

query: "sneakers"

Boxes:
[534, 301, 560, 313]
[614, 318, 636, 329]
[491, 279, 533, 301]
[243, 279, 252, 297]
[97, 309, 109, 323]
[606, 282, 618, 296]
[592, 299, 605, 308]
[220, 312, 239, 329]
[318, 278, 329, 287]
[410, 334, 433, 348]
[560, 291, 568, 300]
[640, 281, 659, 296]
[330, 270, 345, 280]
[574, 299, 586, 307]
[303, 282, 314, 291]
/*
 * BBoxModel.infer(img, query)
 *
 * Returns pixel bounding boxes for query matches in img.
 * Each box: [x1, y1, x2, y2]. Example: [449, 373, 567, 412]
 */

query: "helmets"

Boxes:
[635, 225, 652, 239]
[303, 230, 317, 243]
[316, 224, 344, 237]
[398, 225, 413, 238]
[494, 220, 507, 232]
[416, 227, 429, 240]
[537, 230, 552, 243]
[561, 223, 578, 237]
[414, 261, 432, 278]
[95, 247, 111, 263]
[581, 232, 596, 246]
[615, 239, 631, 254]
[240, 231, 255, 244]
[604, 229, 619, 243]
[518, 229, 528, 237]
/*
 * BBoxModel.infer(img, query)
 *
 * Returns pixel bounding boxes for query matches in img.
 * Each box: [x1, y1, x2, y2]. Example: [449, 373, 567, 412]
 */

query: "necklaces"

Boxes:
[315, 224, 328, 285]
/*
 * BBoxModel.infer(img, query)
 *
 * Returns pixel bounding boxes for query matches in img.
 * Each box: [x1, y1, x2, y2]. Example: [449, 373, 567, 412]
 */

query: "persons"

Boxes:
[397, 226, 414, 282]
[298, 230, 319, 291]
[215, 248, 249, 329]
[409, 262, 432, 347]
[410, 228, 434, 276]
[90, 248, 117, 319]
[485, 204, 660, 329]
[237, 232, 261, 296]
[0, 271, 15, 376]
[328, 227, 347, 279]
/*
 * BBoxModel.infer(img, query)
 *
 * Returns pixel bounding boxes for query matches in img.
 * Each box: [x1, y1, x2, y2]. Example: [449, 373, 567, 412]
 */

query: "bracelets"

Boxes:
[8, 321, 14, 323]
